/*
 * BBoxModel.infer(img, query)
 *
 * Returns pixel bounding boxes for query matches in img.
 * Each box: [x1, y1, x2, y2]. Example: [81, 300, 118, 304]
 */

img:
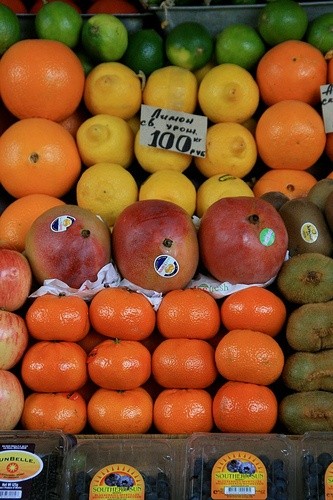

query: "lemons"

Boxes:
[0, 0, 333, 72]
[75, 61, 255, 226]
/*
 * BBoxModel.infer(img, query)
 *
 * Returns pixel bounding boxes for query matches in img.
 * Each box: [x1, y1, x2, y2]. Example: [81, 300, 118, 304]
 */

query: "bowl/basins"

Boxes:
[0, 429, 70, 500]
[185, 432, 298, 500]
[64, 439, 184, 500]
[296, 431, 333, 500]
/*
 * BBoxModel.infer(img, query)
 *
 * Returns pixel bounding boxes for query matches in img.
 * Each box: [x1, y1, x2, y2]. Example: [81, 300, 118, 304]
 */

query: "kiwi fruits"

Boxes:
[258, 179, 333, 433]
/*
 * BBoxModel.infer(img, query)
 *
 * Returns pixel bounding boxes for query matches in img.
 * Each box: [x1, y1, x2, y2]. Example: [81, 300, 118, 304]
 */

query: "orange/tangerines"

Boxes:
[256, 40, 333, 200]
[20, 286, 288, 433]
[0, 39, 82, 252]
